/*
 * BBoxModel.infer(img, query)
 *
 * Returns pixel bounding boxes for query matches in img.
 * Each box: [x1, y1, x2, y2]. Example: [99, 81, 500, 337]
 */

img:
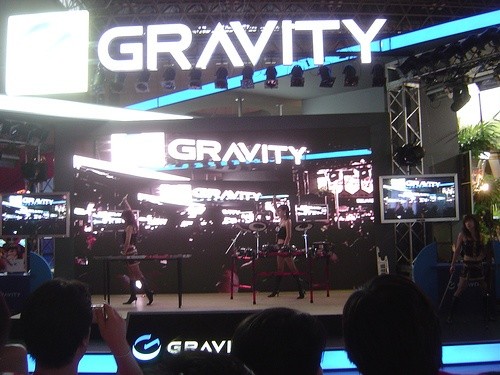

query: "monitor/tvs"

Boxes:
[0, 192, 71, 238]
[379, 173, 460, 224]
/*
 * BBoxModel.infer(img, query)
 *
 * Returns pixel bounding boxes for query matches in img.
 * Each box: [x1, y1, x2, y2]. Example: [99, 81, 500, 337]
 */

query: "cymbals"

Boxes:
[248, 221, 266, 233]
[294, 221, 313, 231]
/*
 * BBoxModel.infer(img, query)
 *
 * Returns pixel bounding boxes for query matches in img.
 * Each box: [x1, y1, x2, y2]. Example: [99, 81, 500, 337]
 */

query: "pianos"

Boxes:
[90, 252, 192, 303]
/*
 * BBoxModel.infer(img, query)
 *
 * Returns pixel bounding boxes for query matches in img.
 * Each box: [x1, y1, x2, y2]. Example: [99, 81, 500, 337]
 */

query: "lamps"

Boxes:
[318, 64, 336, 88]
[9, 122, 48, 145]
[160, 61, 177, 91]
[135, 69, 151, 93]
[371, 63, 388, 87]
[342, 65, 359, 87]
[290, 65, 305, 87]
[240, 66, 255, 90]
[264, 65, 279, 89]
[396, 26, 500, 112]
[190, 67, 203, 90]
[90, 60, 107, 97]
[108, 69, 127, 94]
[214, 62, 228, 90]
[396, 143, 426, 167]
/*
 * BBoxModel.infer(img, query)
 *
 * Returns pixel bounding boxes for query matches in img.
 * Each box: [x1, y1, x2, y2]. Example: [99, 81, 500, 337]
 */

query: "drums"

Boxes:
[277, 242, 297, 255]
[234, 245, 259, 258]
[259, 241, 281, 253]
[311, 242, 334, 257]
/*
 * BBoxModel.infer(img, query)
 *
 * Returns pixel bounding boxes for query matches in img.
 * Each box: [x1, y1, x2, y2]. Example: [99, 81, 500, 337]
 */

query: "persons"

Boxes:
[449, 214, 489, 292]
[267, 192, 305, 300]
[122, 196, 157, 305]
[0, 275, 456, 375]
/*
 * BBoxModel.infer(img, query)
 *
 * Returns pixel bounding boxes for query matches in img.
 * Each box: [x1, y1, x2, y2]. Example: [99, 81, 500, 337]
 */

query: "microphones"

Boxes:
[236, 225, 252, 234]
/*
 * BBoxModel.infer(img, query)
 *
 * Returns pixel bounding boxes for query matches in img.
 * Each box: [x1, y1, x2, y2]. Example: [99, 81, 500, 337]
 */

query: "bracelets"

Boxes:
[114, 349, 131, 359]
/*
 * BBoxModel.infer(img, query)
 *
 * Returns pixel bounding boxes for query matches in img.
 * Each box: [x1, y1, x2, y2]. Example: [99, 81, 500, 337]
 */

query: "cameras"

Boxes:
[90, 304, 105, 322]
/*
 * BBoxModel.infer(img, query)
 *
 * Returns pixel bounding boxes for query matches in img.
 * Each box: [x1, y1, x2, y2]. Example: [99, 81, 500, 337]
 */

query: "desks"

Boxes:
[93, 254, 192, 307]
[230, 252, 334, 305]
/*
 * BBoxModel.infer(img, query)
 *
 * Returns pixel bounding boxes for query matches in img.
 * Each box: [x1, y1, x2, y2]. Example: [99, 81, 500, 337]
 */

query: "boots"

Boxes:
[295, 275, 307, 299]
[484, 297, 497, 321]
[268, 276, 282, 297]
[140, 277, 156, 305]
[447, 296, 459, 324]
[123, 280, 137, 304]
